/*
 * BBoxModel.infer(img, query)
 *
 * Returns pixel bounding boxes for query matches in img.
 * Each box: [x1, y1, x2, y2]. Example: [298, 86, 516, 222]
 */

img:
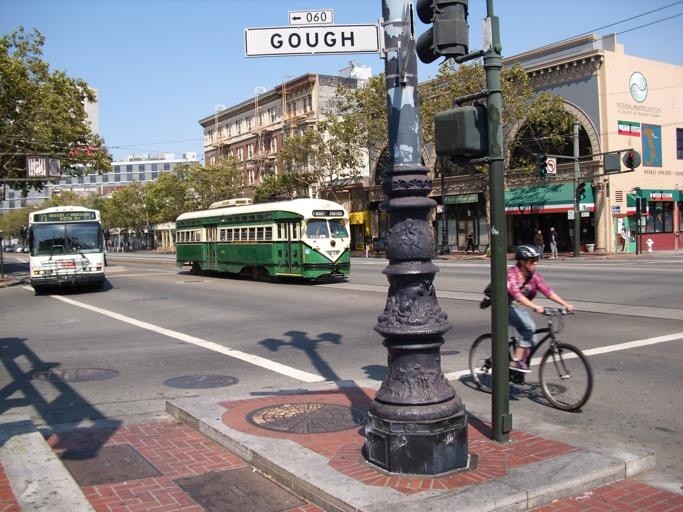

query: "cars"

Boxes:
[4, 247, 29, 253]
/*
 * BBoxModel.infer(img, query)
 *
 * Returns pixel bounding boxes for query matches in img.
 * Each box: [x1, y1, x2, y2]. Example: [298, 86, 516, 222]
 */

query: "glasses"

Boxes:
[523, 259, 538, 265]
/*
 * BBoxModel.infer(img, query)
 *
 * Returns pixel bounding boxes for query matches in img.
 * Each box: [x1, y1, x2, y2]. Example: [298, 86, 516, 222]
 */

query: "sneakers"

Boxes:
[538, 256, 558, 259]
[509, 381, 532, 391]
[509, 359, 532, 374]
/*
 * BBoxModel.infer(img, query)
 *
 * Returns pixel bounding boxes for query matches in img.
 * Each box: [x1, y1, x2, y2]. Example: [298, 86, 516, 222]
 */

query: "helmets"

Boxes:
[514, 244, 540, 258]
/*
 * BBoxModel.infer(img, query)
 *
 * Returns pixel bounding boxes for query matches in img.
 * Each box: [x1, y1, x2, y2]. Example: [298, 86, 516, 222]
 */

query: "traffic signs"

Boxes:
[242, 9, 382, 57]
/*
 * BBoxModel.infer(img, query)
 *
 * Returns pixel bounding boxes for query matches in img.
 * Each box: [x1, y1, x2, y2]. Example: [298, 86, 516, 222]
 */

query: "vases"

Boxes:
[585, 244, 596, 252]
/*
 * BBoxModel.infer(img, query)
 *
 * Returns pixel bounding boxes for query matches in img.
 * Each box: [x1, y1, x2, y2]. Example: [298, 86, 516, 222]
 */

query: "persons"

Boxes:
[484, 244, 575, 373]
[620, 223, 625, 251]
[655, 211, 663, 225]
[464, 229, 475, 255]
[534, 229, 546, 260]
[548, 227, 559, 259]
[120, 241, 124, 250]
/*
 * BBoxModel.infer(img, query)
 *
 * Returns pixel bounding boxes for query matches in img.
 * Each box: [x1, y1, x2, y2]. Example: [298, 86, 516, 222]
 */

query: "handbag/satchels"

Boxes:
[485, 282, 512, 305]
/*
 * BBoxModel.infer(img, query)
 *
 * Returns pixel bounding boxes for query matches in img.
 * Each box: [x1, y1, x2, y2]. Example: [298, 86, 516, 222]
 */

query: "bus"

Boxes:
[20, 206, 109, 293]
[174, 198, 349, 286]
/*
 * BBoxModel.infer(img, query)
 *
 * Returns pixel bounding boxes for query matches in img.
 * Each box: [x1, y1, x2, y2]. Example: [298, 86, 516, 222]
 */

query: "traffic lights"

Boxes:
[628, 152, 635, 169]
[578, 182, 585, 201]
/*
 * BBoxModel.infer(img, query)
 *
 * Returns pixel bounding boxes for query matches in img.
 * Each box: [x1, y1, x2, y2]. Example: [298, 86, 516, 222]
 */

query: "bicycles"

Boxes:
[467, 308, 593, 412]
